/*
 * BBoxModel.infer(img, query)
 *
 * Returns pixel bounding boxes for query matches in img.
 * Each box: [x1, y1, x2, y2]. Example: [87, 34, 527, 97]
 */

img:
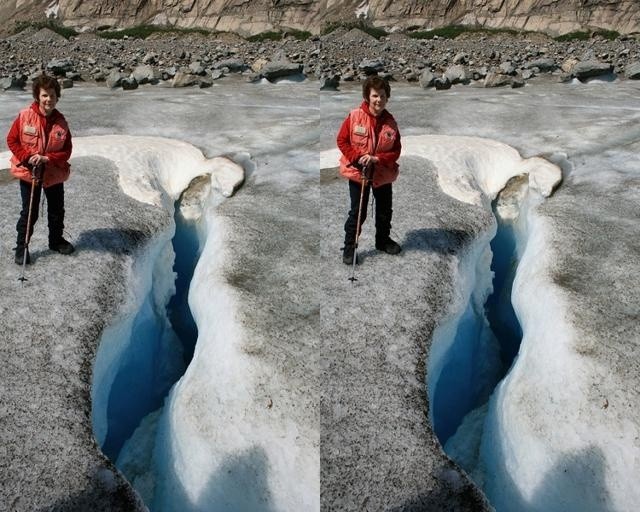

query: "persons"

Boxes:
[6, 72, 77, 266]
[337, 74, 403, 267]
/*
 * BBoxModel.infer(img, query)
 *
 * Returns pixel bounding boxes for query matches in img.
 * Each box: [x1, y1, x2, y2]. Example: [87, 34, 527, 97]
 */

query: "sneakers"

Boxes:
[339, 239, 359, 266]
[12, 240, 31, 266]
[48, 236, 74, 255]
[374, 235, 403, 255]
